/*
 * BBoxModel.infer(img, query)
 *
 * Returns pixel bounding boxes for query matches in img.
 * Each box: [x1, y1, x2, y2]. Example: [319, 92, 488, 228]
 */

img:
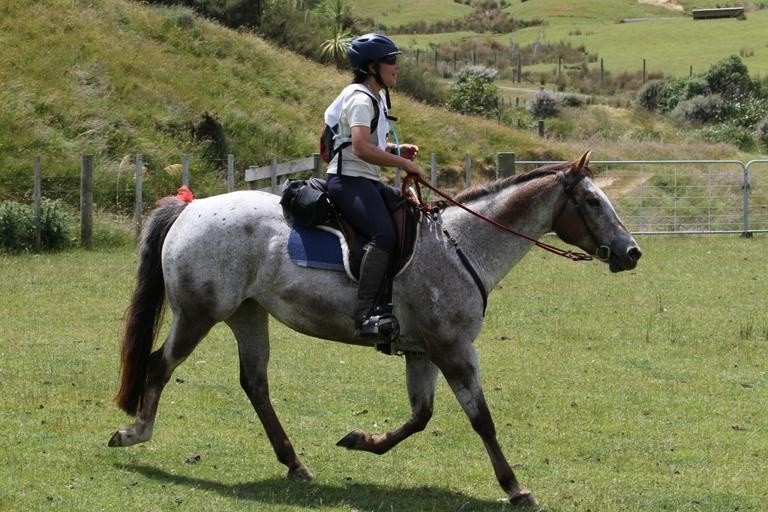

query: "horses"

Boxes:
[107, 147, 644, 511]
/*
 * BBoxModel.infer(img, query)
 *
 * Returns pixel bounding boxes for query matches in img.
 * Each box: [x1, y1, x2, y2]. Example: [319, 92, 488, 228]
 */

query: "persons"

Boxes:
[319, 32, 425, 341]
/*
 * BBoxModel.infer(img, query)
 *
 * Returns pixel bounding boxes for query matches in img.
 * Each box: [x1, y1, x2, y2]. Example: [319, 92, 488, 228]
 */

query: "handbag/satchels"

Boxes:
[282, 178, 335, 228]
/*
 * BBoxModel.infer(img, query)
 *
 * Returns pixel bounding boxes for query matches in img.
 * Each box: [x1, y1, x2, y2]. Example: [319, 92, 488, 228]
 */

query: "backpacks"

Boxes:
[320, 89, 397, 163]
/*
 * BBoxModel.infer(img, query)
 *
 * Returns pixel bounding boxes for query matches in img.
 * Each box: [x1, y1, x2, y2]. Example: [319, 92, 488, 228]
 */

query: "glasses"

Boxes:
[378, 55, 396, 65]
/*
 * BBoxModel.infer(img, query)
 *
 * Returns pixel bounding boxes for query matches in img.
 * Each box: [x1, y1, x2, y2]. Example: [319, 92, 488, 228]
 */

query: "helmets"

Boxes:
[347, 33, 403, 71]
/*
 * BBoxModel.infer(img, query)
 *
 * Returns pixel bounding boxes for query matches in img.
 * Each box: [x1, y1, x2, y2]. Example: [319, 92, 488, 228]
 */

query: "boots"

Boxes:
[354, 242, 397, 335]
